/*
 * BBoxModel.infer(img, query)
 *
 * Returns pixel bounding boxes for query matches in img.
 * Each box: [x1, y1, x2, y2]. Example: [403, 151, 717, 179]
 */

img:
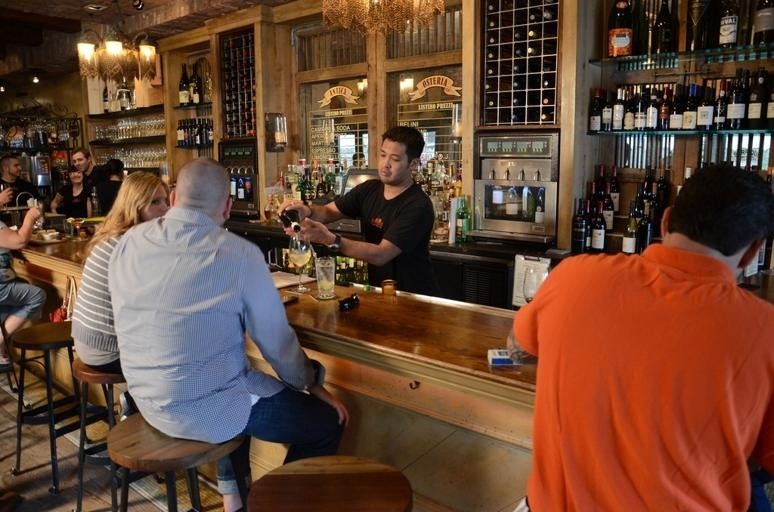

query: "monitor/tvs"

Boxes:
[128, 168, 162, 177]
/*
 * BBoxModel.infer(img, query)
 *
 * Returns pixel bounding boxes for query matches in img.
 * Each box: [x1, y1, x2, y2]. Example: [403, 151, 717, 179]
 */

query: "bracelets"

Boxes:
[306, 204, 313, 218]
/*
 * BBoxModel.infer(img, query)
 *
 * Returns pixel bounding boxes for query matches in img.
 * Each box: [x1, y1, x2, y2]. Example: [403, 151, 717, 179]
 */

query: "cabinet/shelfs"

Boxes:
[587, 41, 774, 135]
[88, 103, 166, 175]
[172, 101, 213, 148]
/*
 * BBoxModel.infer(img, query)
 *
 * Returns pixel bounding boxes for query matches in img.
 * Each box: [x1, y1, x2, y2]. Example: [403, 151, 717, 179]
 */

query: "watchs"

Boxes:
[327, 232, 341, 253]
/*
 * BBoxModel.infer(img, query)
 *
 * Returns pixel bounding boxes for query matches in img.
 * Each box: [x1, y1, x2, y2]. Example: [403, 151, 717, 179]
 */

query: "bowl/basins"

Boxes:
[37, 229, 56, 241]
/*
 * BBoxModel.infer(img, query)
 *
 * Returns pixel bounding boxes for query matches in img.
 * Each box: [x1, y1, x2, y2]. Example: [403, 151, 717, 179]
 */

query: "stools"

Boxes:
[12, 321, 107, 496]
[107, 413, 242, 512]
[246, 455, 413, 512]
[71, 357, 126, 512]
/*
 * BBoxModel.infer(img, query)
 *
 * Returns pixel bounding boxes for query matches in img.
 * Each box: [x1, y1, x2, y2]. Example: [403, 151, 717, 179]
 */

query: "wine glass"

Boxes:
[94, 117, 165, 169]
[289, 235, 314, 294]
[66, 216, 85, 237]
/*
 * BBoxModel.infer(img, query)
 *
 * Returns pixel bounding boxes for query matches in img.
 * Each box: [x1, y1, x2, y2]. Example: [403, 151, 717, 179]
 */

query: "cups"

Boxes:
[312, 256, 338, 299]
[521, 266, 550, 304]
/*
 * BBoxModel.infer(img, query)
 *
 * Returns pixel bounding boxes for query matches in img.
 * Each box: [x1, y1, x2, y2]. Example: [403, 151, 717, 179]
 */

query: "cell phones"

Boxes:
[281, 295, 298, 305]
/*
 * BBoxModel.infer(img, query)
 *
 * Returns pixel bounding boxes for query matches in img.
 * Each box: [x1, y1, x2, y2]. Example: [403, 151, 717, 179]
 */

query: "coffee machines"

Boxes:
[32, 155, 51, 205]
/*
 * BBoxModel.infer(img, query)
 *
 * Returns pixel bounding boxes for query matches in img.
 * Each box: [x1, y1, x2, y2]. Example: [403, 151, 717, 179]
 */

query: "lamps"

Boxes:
[323, 1, 446, 37]
[77, 0, 156, 83]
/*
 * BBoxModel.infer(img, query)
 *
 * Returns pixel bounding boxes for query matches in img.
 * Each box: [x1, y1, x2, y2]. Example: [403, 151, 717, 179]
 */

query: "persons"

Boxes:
[51, 147, 124, 218]
[70, 170, 170, 416]
[276, 125, 435, 296]
[0, 155, 44, 207]
[0, 208, 47, 365]
[353, 154, 364, 167]
[108, 156, 349, 512]
[507, 162, 774, 512]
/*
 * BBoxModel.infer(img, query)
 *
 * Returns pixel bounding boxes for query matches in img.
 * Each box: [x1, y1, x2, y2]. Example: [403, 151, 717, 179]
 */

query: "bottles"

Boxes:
[264, 165, 336, 221]
[176, 117, 213, 147]
[588, 67, 773, 133]
[334, 256, 370, 285]
[485, 4, 556, 124]
[491, 185, 504, 215]
[23, 127, 47, 148]
[521, 189, 531, 219]
[580, 162, 691, 255]
[412, 161, 471, 243]
[504, 186, 518, 218]
[230, 167, 252, 201]
[119, 75, 131, 111]
[605, 0, 773, 70]
[178, 60, 201, 107]
[101, 79, 112, 112]
[277, 206, 301, 232]
[533, 188, 546, 224]
